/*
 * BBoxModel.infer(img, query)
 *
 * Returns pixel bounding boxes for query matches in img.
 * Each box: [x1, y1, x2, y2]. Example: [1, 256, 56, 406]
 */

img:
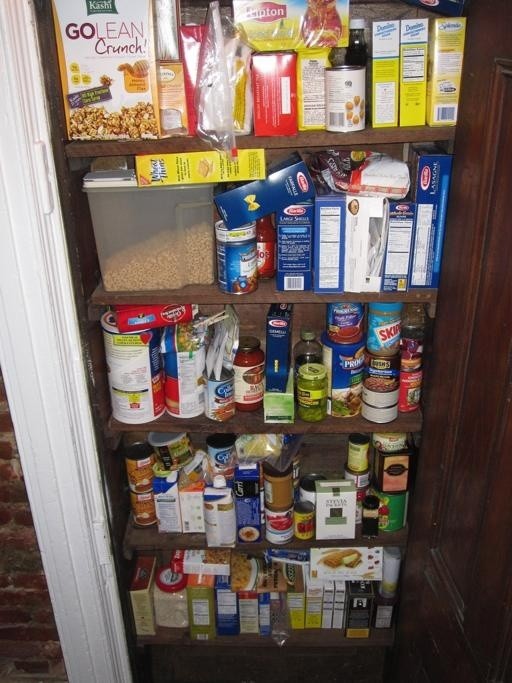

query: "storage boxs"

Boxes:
[81, 164, 219, 293]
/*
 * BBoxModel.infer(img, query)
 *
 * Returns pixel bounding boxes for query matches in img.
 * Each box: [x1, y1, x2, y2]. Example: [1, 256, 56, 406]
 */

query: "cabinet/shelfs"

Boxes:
[29, 0, 471, 650]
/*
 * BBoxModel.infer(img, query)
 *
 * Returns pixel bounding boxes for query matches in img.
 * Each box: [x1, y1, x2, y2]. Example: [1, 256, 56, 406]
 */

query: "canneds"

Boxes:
[124, 431, 418, 545]
[100, 301, 425, 425]
[214, 219, 257, 294]
[324, 63, 366, 132]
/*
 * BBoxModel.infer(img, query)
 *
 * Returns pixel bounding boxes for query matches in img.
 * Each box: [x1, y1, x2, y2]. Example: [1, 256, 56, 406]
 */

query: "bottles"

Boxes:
[256, 216, 276, 280]
[346, 18, 369, 66]
[293, 330, 323, 383]
